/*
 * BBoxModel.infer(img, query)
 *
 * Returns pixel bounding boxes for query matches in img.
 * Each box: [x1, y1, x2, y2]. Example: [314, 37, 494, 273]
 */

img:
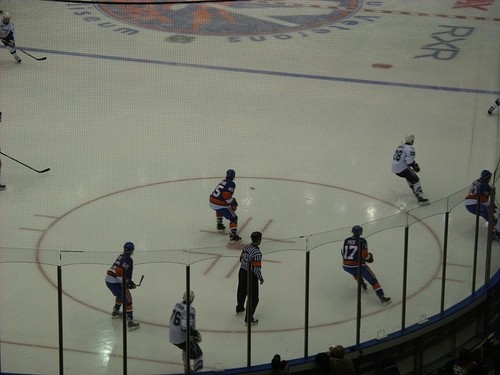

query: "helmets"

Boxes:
[3, 12, 11, 19]
[183, 289, 195, 303]
[251, 231, 262, 240]
[352, 225, 362, 235]
[226, 169, 235, 175]
[480, 170, 492, 177]
[124, 242, 135, 251]
[406, 134, 416, 143]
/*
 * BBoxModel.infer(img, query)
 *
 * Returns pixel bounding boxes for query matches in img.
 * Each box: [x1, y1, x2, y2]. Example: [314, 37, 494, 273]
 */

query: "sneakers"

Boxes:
[379, 296, 392, 305]
[361, 282, 368, 292]
[127, 320, 139, 331]
[236, 306, 246, 314]
[229, 228, 242, 243]
[417, 197, 429, 206]
[217, 224, 225, 232]
[488, 107, 495, 115]
[15, 56, 22, 63]
[111, 308, 123, 318]
[244, 316, 258, 324]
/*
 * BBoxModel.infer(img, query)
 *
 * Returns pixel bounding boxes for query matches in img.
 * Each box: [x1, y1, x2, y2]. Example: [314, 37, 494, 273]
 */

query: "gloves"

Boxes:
[259, 278, 264, 284]
[191, 329, 202, 342]
[487, 205, 498, 215]
[412, 163, 420, 172]
[365, 253, 373, 263]
[127, 280, 136, 289]
[230, 198, 238, 210]
[10, 40, 15, 48]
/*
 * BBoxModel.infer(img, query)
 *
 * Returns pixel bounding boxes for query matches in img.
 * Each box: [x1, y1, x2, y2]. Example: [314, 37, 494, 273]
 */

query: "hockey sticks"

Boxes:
[0, 38, 47, 61]
[0, 152, 51, 175]
[134, 274, 144, 286]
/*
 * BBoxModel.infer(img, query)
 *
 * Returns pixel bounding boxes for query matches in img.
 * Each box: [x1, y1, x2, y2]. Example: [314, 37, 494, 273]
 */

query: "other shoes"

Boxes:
[492, 230, 500, 242]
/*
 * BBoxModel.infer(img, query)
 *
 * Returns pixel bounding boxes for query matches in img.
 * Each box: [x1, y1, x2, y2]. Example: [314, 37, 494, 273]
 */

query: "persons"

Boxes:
[0, 12, 21, 63]
[315, 345, 357, 375]
[236, 232, 264, 325]
[467, 170, 500, 242]
[103, 241, 146, 332]
[487, 97, 500, 116]
[392, 134, 429, 205]
[0, 112, 6, 190]
[168, 291, 204, 371]
[207, 169, 243, 244]
[342, 226, 391, 304]
[271, 355, 291, 375]
